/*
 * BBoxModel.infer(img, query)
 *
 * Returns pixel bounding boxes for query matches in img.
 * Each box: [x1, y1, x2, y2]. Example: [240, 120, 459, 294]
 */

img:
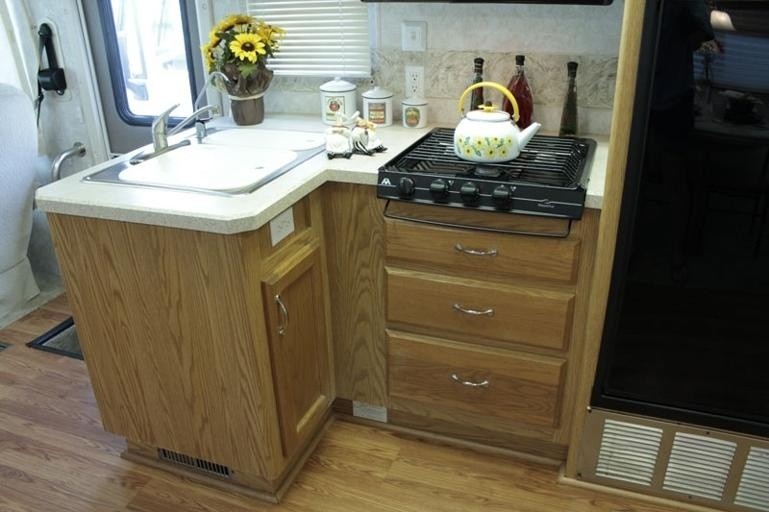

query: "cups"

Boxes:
[318, 77, 429, 130]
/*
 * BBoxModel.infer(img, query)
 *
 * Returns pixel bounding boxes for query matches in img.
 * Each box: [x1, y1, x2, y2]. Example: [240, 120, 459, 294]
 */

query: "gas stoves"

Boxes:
[375, 125, 599, 221]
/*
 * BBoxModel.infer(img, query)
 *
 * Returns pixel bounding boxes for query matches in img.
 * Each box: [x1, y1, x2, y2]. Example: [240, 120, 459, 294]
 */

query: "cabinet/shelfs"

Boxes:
[383, 200, 601, 472]
[252, 188, 338, 506]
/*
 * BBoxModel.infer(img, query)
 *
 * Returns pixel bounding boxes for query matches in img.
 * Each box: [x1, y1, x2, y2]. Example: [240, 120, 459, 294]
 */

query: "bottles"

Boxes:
[470, 57, 579, 136]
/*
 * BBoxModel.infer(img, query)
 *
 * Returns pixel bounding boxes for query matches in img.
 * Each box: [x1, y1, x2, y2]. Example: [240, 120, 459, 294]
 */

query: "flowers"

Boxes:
[199, 14, 287, 93]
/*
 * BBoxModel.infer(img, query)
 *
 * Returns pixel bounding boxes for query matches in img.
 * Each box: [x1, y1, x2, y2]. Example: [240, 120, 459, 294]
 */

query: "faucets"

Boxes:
[150, 103, 179, 149]
[194, 71, 230, 137]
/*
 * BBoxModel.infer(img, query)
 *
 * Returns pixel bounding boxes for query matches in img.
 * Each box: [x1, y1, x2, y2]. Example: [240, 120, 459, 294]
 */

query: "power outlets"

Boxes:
[405, 66, 424, 98]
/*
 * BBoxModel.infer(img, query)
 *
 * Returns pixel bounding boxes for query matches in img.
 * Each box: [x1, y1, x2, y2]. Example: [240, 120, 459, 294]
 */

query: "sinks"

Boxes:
[81, 146, 298, 202]
[191, 125, 330, 151]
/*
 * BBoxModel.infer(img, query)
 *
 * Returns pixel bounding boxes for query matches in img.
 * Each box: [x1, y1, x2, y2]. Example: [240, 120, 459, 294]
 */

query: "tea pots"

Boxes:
[453, 82, 542, 162]
